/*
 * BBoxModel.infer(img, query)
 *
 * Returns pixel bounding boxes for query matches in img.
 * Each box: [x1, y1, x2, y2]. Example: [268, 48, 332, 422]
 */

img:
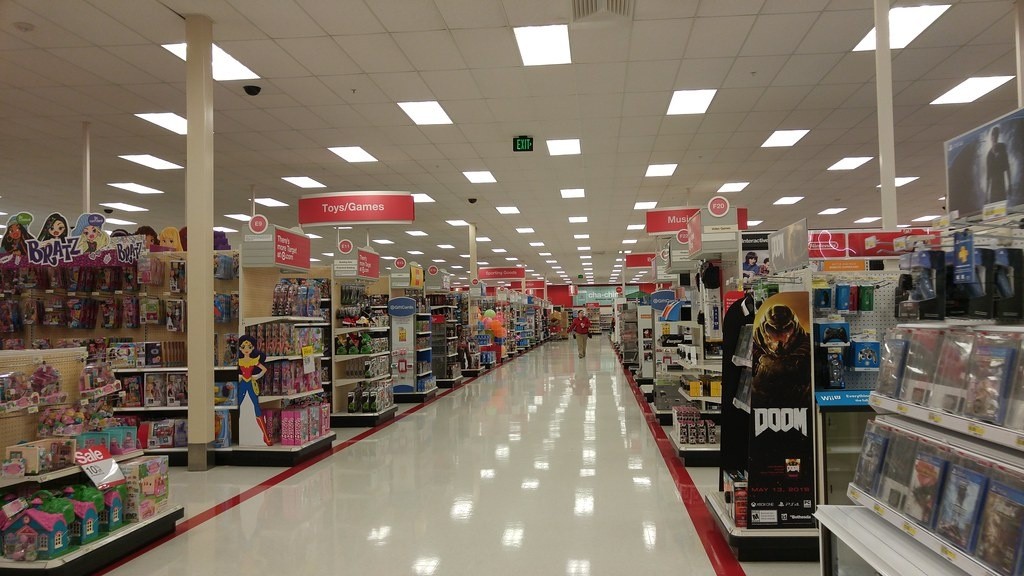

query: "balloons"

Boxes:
[477, 308, 507, 356]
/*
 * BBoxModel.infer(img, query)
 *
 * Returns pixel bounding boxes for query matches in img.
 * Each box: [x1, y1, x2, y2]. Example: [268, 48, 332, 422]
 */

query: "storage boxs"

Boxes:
[653, 343, 717, 445]
[417, 309, 494, 392]
[105, 346, 136, 369]
[245, 322, 323, 356]
[347, 386, 363, 413]
[215, 380, 238, 406]
[261, 402, 331, 446]
[343, 317, 390, 353]
[144, 372, 167, 407]
[166, 372, 188, 407]
[370, 386, 385, 413]
[208, 409, 232, 448]
[256, 360, 322, 396]
[378, 379, 394, 409]
[361, 387, 375, 413]
[147, 419, 174, 448]
[173, 419, 188, 447]
[357, 380, 379, 388]
[344, 355, 389, 379]
[117, 456, 169, 522]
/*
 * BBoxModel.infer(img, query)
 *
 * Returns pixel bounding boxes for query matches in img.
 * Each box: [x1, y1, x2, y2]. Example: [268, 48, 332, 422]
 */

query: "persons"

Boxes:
[456, 325, 471, 370]
[565, 310, 591, 359]
[751, 304, 811, 409]
[743, 252, 770, 275]
[984, 126, 1012, 204]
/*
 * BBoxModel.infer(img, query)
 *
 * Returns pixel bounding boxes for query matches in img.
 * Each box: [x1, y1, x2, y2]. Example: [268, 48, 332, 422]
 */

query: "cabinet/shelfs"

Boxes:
[431, 305, 464, 389]
[479, 344, 496, 370]
[668, 320, 722, 467]
[0, 450, 185, 576]
[112, 366, 238, 467]
[243, 315, 337, 468]
[811, 322, 1024, 576]
[394, 313, 439, 404]
[507, 302, 613, 359]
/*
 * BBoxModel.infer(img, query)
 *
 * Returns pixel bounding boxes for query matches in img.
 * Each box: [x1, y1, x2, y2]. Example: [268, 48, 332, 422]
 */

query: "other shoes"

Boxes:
[583, 353, 585, 357]
[578, 354, 583, 358]
